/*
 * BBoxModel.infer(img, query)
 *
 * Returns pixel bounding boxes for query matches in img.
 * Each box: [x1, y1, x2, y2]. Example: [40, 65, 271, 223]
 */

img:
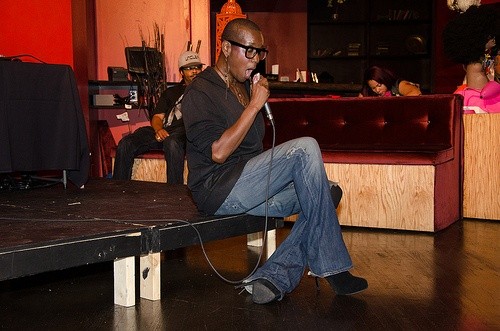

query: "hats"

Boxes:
[179, 50, 205, 69]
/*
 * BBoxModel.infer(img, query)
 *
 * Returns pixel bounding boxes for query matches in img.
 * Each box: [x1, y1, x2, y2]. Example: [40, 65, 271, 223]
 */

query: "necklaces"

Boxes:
[215, 64, 248, 107]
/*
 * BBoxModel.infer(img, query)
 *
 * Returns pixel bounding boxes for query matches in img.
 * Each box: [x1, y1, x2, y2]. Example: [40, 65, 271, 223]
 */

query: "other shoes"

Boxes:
[168, 182, 184, 192]
[96, 178, 133, 187]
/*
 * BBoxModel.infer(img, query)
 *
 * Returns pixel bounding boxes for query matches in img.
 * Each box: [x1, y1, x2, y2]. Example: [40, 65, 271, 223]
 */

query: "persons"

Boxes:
[179, 19, 369, 304]
[112, 51, 205, 183]
[359, 66, 422, 98]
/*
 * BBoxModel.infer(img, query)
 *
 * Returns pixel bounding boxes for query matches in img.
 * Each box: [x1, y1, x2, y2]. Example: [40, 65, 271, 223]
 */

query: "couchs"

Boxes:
[111, 94, 466, 232]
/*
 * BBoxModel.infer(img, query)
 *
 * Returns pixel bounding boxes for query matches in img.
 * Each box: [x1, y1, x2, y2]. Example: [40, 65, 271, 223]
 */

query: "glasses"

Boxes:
[227, 40, 270, 60]
[181, 65, 203, 70]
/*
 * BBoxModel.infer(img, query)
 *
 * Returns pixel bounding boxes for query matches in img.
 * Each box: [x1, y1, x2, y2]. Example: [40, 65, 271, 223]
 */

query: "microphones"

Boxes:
[253, 72, 273, 120]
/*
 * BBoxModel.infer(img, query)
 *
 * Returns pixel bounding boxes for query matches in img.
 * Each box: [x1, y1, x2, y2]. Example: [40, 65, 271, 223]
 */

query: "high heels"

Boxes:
[315, 270, 368, 296]
[234, 279, 279, 304]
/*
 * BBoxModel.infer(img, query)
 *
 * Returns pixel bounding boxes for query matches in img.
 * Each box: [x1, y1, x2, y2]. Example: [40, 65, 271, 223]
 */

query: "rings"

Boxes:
[157, 137, 160, 140]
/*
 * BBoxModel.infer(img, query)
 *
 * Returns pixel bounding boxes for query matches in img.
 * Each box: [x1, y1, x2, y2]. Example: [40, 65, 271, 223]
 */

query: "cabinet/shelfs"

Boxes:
[306, 0, 437, 95]
